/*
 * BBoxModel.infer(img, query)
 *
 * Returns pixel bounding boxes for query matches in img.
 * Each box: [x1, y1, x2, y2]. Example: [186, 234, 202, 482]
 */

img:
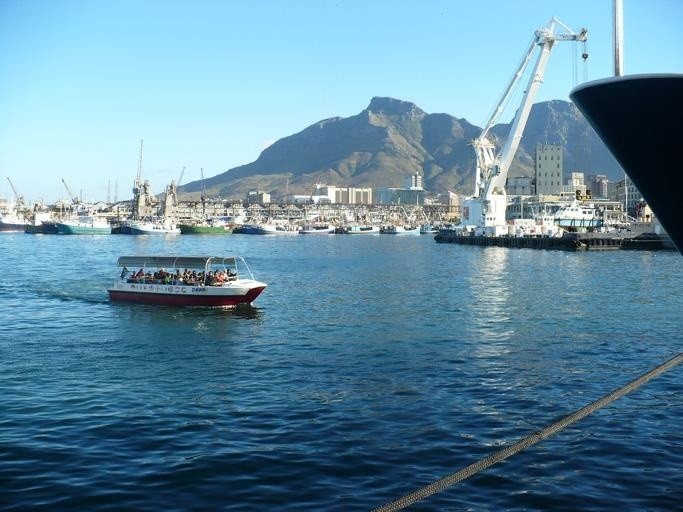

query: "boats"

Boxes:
[551, 200, 601, 229]
[434, 18, 589, 252]
[108, 256, 266, 307]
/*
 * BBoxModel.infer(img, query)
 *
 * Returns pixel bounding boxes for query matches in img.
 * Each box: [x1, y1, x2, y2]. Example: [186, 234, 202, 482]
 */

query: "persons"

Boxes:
[120, 267, 237, 287]
